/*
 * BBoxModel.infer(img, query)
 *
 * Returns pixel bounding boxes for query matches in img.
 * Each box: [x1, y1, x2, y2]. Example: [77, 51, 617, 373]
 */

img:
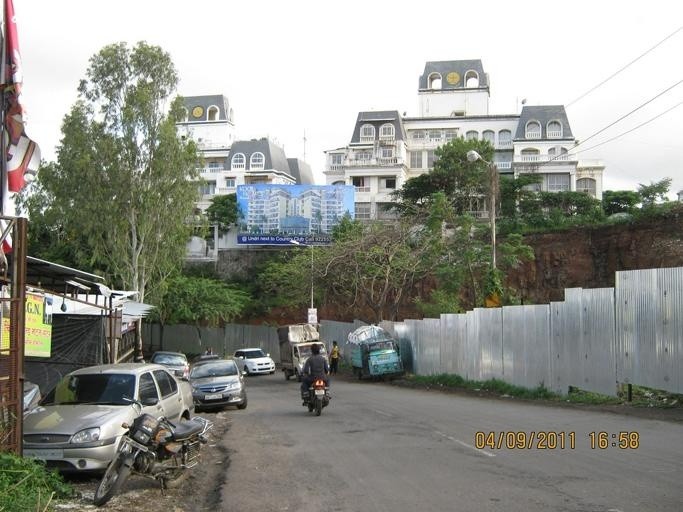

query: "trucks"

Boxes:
[280, 343, 331, 382]
[351, 331, 404, 382]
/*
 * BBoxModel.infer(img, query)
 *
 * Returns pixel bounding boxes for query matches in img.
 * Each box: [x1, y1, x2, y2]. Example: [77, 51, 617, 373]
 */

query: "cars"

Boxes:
[149, 350, 191, 381]
[23, 362, 188, 471]
[188, 359, 247, 412]
[233, 348, 276, 376]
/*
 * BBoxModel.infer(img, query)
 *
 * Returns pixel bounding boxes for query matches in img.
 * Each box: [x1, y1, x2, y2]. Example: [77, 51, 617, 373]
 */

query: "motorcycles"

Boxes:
[93, 394, 212, 504]
[301, 377, 330, 417]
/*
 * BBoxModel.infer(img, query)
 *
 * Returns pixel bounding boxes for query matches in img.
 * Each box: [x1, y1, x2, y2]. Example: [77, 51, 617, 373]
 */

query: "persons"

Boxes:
[325, 344, 331, 364]
[328, 341, 340, 376]
[205, 346, 213, 355]
[300, 344, 332, 398]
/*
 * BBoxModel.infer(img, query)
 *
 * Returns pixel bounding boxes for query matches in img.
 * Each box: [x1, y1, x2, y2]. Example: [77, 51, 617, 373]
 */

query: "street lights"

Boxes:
[290, 238, 315, 308]
[467, 151, 497, 272]
